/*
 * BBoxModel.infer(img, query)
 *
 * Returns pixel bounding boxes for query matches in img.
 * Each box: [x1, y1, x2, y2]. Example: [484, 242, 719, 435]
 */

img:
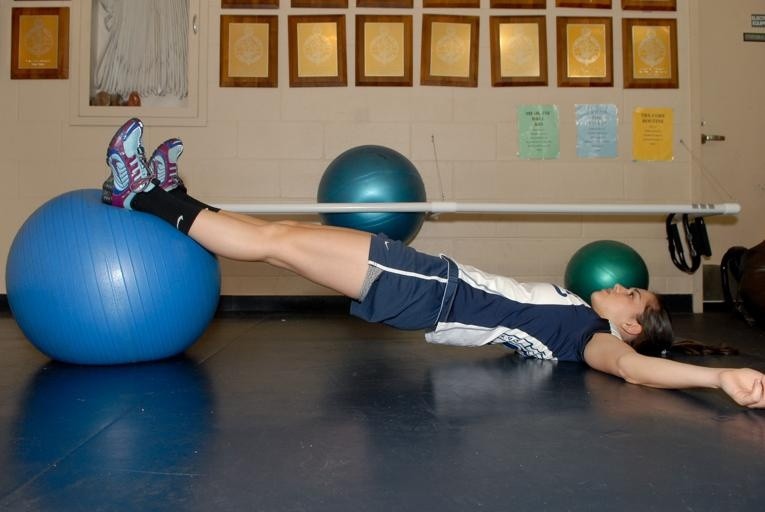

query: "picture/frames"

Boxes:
[292, 0, 348, 8]
[556, 0, 611, 7]
[355, 15, 413, 86]
[622, 17, 679, 89]
[420, 14, 479, 87]
[221, 1, 278, 8]
[423, 1, 480, 8]
[556, 16, 613, 86]
[221, 15, 279, 88]
[622, 1, 677, 10]
[10, 6, 68, 81]
[489, 15, 547, 87]
[490, 1, 546, 9]
[356, 1, 414, 8]
[288, 14, 346, 86]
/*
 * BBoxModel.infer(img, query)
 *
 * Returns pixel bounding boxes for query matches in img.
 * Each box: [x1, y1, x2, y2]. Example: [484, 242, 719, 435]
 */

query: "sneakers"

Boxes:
[147, 138, 184, 192]
[100, 117, 161, 212]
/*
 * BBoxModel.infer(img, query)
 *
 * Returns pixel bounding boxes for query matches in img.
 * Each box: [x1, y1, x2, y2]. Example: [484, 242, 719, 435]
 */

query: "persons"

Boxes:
[101, 116, 765, 410]
